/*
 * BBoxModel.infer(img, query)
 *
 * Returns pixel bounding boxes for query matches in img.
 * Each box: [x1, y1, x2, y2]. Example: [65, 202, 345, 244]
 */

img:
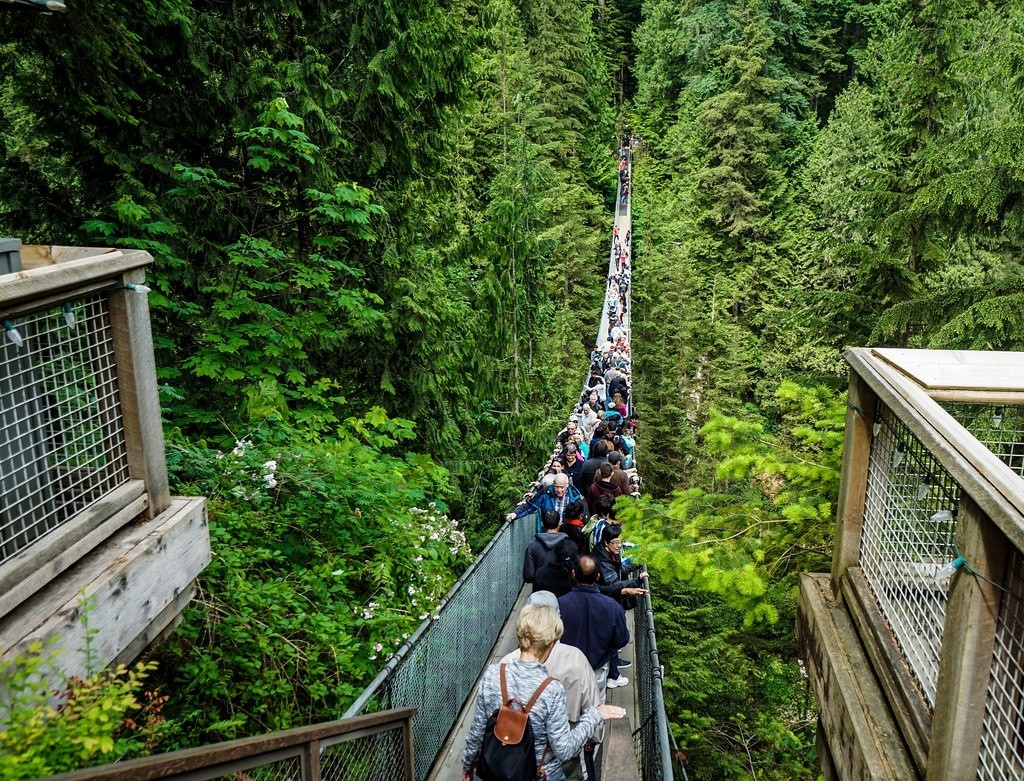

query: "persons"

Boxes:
[461, 131, 640, 781]
[557, 556, 630, 759]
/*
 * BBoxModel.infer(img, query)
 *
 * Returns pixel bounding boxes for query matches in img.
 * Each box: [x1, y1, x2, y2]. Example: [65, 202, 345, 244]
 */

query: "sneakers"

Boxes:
[606, 674, 629, 688]
[617, 658, 632, 669]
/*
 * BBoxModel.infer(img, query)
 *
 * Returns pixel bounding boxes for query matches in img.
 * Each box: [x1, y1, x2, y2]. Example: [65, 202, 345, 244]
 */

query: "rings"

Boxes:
[612, 710, 615, 714]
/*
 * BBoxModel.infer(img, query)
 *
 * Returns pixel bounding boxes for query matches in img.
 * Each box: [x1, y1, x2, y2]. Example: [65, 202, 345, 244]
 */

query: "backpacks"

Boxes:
[475, 663, 559, 781]
[543, 542, 572, 597]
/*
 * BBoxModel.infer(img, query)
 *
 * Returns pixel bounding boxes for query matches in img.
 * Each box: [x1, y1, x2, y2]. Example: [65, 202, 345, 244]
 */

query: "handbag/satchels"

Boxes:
[623, 593, 637, 610]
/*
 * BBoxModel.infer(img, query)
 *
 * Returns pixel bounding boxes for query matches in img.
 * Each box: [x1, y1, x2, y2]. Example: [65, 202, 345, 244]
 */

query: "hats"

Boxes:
[609, 451, 622, 465]
[570, 415, 578, 422]
[527, 590, 561, 618]
[608, 402, 616, 409]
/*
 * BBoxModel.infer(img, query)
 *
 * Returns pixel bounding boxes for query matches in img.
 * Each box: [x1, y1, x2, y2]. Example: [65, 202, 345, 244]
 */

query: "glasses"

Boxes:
[553, 458, 562, 462]
[609, 540, 621, 546]
[573, 421, 578, 423]
